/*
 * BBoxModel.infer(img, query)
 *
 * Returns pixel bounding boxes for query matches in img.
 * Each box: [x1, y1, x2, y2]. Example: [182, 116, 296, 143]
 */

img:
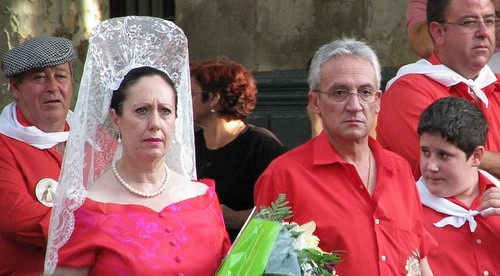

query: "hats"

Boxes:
[2, 35, 75, 78]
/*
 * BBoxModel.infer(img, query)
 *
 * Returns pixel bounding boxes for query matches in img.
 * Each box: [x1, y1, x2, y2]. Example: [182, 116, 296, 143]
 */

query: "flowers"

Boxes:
[258, 193, 346, 276]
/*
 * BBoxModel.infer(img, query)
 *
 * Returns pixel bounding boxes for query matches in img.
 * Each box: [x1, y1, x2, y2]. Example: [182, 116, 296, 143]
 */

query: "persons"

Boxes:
[375, 0, 500, 181]
[254, 37, 433, 276]
[306, 57, 376, 141]
[407, 0, 500, 59]
[416, 96, 500, 276]
[189, 57, 285, 245]
[42, 15, 232, 276]
[0, 35, 117, 276]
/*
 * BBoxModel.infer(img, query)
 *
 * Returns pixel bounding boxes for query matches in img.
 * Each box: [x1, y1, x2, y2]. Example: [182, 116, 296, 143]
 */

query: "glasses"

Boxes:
[313, 84, 379, 103]
[191, 86, 222, 97]
[438, 16, 499, 31]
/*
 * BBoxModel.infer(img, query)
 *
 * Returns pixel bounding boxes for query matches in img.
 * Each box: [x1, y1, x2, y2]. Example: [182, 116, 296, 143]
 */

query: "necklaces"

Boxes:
[113, 160, 169, 197]
[366, 149, 371, 190]
[207, 120, 241, 150]
